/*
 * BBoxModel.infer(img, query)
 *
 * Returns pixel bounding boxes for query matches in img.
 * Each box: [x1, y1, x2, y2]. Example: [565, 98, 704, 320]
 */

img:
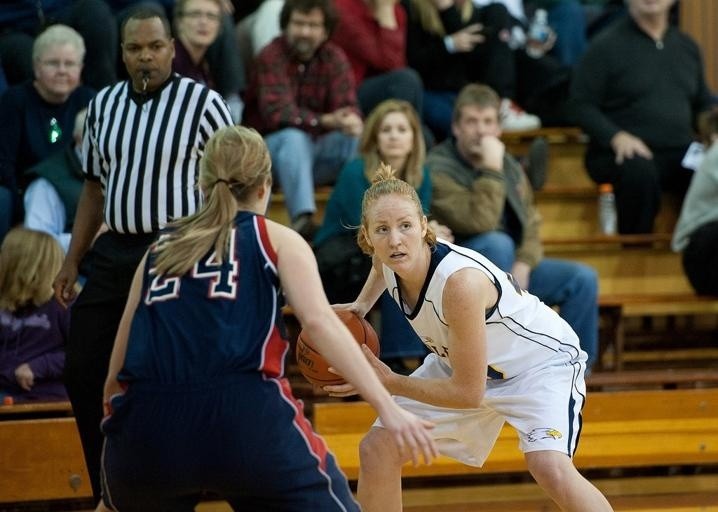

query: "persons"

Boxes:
[52, 11, 236, 512]
[0, 2, 717, 418]
[328, 161, 614, 512]
[101, 126, 440, 512]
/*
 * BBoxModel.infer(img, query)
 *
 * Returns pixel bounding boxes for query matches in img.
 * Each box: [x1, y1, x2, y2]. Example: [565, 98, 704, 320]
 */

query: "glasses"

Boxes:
[182, 10, 222, 20]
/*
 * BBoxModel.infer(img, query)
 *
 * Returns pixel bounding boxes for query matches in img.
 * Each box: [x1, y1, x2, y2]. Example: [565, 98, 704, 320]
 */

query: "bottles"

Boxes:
[598, 183, 618, 235]
[525, 9, 547, 59]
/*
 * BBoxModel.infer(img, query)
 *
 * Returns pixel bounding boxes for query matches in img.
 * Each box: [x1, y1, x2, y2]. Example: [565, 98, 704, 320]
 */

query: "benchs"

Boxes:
[0, 127, 718, 512]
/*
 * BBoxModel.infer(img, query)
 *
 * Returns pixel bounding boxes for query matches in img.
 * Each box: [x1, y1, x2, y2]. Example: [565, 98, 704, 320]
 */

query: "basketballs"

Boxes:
[296, 310, 381, 392]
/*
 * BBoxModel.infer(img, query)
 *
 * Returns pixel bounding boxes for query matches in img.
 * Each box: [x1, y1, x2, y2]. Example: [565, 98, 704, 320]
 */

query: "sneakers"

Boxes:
[499, 98, 540, 135]
